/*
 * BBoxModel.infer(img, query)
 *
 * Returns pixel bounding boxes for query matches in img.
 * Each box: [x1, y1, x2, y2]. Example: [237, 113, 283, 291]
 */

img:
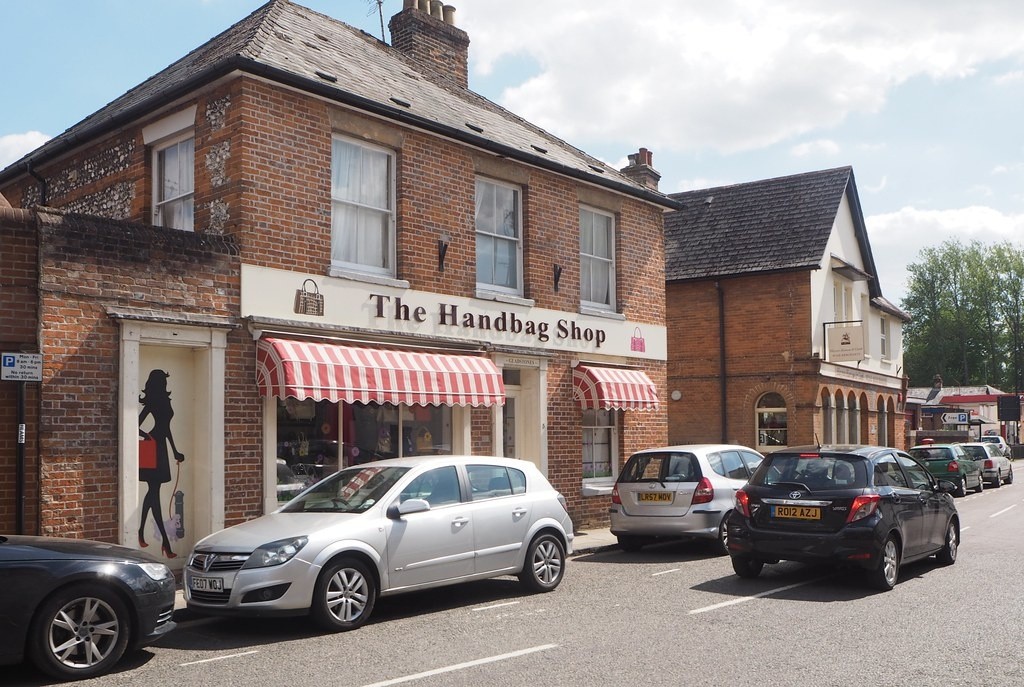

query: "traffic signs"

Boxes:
[941, 412, 969, 425]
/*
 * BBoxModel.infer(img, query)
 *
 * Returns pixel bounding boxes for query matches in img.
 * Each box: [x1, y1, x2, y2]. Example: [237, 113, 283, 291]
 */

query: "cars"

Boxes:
[608, 443, 765, 555]
[182, 454, 574, 632]
[0, 534, 177, 681]
[907, 442, 1013, 497]
[726, 443, 960, 591]
[277, 458, 339, 506]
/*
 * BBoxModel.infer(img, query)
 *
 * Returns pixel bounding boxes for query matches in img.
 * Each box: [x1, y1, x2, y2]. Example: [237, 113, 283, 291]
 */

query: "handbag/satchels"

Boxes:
[417, 426, 433, 453]
[289, 464, 319, 498]
[290, 431, 308, 456]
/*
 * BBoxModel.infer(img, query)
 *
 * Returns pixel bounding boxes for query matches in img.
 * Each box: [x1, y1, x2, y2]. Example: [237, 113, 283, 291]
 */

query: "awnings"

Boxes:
[252, 328, 507, 406]
[572, 358, 660, 409]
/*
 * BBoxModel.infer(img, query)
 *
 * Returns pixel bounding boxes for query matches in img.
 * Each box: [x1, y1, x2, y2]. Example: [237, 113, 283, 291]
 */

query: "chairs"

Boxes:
[488, 477, 510, 491]
[673, 461, 692, 476]
[833, 465, 852, 483]
[919, 452, 930, 457]
[804, 460, 828, 477]
[424, 473, 457, 503]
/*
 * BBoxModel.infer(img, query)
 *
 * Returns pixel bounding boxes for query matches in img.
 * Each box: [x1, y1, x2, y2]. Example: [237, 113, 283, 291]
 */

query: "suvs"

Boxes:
[977, 436, 1011, 460]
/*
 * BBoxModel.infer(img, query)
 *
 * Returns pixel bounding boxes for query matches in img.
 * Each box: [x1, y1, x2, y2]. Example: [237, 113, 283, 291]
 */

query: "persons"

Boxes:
[805, 462, 828, 478]
[835, 464, 854, 483]
